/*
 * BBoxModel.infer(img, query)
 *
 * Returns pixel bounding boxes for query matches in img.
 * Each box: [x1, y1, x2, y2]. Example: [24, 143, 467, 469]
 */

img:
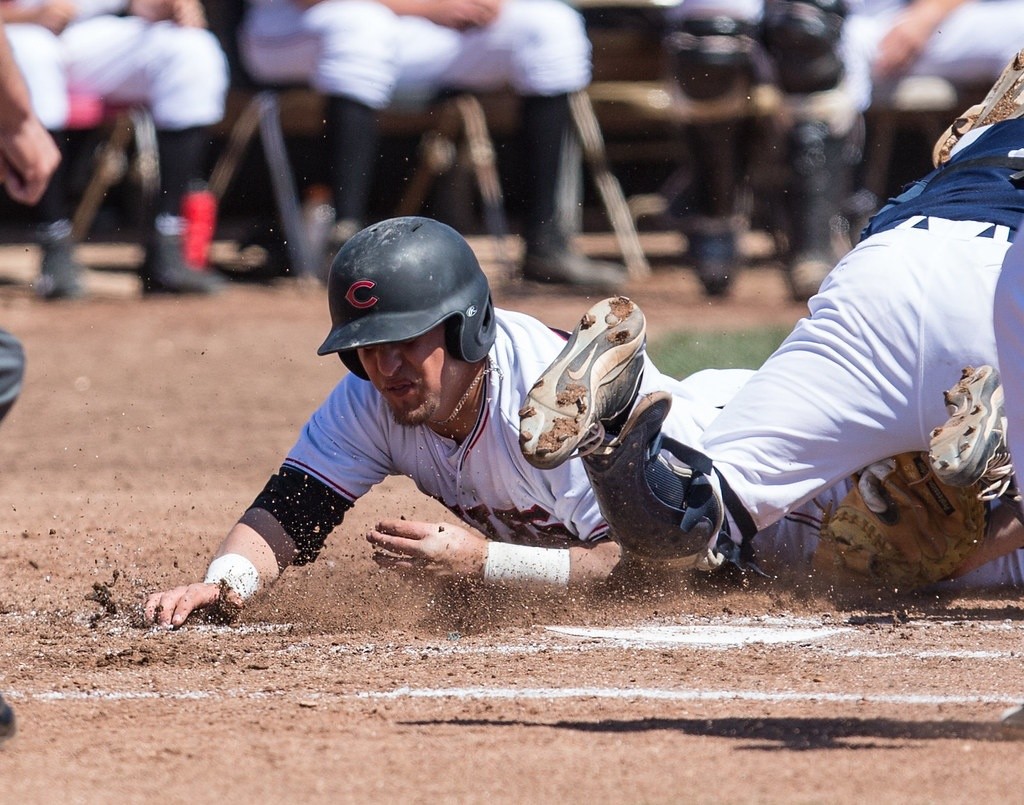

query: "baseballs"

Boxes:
[857, 459, 897, 514]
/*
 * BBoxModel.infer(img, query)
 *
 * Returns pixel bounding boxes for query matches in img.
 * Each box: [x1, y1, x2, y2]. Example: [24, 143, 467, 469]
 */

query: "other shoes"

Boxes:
[691, 234, 738, 291]
[523, 251, 631, 293]
[791, 249, 836, 298]
[317, 221, 357, 279]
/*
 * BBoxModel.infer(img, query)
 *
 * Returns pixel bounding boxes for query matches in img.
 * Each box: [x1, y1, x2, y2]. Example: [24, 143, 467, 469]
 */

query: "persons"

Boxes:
[239, 0, 626, 293]
[655, 0, 1024, 300]
[519, 50, 1024, 565]
[134, 214, 1024, 629]
[0, 0, 225, 408]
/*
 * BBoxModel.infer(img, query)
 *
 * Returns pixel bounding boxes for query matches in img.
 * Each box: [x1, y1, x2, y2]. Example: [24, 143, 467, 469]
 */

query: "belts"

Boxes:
[859, 216, 1017, 244]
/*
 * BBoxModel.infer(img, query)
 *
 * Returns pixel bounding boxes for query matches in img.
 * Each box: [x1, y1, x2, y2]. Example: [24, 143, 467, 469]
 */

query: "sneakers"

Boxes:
[519, 296, 647, 470]
[928, 365, 1022, 502]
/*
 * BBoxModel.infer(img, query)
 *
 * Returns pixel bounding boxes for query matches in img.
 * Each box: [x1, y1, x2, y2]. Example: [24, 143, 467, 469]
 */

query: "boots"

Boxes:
[143, 233, 210, 291]
[37, 232, 78, 297]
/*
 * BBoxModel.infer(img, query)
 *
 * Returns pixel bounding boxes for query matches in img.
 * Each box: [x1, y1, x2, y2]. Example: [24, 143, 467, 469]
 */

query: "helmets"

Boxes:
[317, 216, 496, 381]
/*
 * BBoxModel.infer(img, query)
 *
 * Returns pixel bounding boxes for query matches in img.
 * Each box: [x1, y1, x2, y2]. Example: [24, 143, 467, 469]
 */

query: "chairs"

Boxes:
[42, 0, 960, 284]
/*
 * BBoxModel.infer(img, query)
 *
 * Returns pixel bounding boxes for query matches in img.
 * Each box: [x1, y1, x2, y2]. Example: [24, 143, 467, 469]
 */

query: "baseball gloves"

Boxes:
[811, 451, 991, 594]
